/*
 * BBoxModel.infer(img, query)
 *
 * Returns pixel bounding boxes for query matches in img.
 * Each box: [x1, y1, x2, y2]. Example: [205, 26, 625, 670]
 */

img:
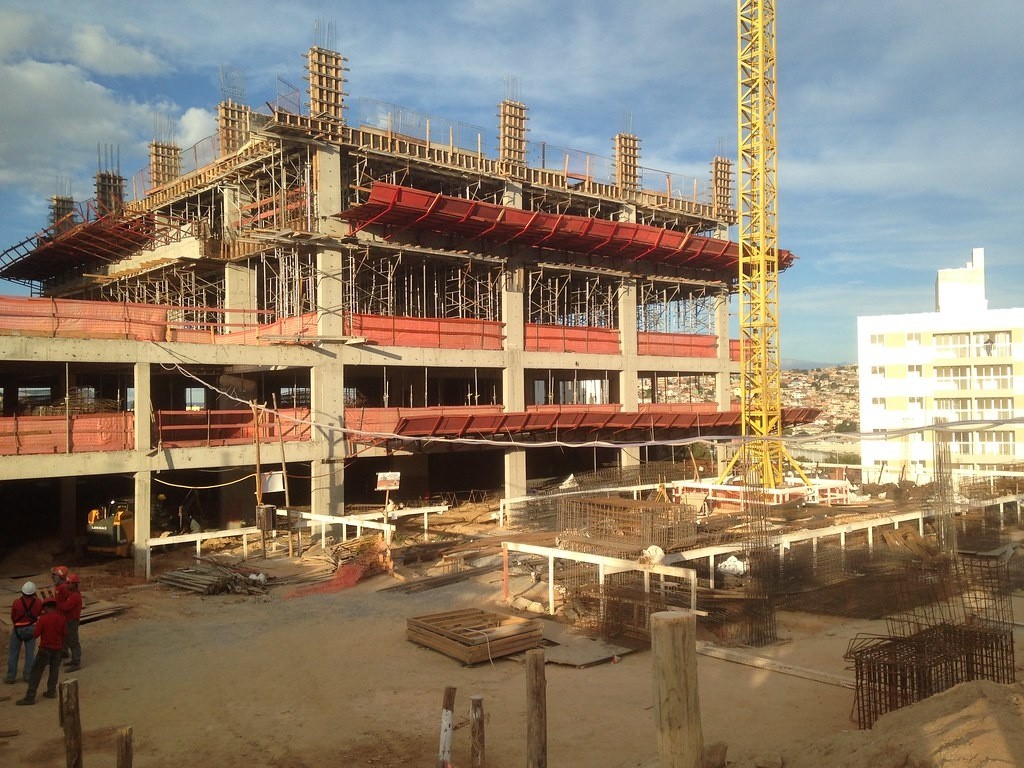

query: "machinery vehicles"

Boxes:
[85, 494, 178, 557]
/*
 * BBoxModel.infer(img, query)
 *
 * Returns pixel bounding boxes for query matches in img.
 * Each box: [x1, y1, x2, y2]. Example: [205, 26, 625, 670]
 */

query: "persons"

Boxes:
[51, 566, 69, 599]
[15, 597, 69, 705]
[3, 581, 46, 683]
[57, 574, 82, 673]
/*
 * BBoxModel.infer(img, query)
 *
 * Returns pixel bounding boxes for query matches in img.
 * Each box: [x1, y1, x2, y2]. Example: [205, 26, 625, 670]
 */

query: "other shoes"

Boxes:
[65, 666, 81, 673]
[24, 679, 29, 682]
[43, 692, 56, 698]
[16, 699, 34, 705]
[3, 677, 15, 684]
[63, 661, 80, 666]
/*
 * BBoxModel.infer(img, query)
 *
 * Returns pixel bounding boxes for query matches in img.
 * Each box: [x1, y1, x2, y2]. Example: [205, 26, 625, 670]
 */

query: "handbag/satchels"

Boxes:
[14, 622, 33, 641]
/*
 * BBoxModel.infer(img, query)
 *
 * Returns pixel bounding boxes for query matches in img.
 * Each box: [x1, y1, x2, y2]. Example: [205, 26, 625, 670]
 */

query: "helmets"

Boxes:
[66, 574, 80, 583]
[51, 566, 68, 580]
[21, 581, 36, 595]
[41, 597, 57, 606]
